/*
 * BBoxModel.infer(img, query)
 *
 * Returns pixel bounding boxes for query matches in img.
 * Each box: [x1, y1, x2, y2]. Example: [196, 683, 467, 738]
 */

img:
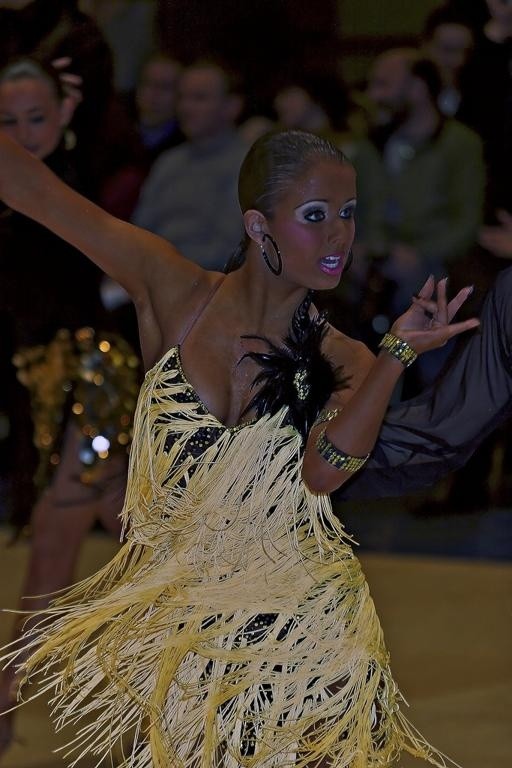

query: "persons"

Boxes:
[0, 328, 143, 768]
[1, 121, 485, 768]
[1, 1, 512, 534]
